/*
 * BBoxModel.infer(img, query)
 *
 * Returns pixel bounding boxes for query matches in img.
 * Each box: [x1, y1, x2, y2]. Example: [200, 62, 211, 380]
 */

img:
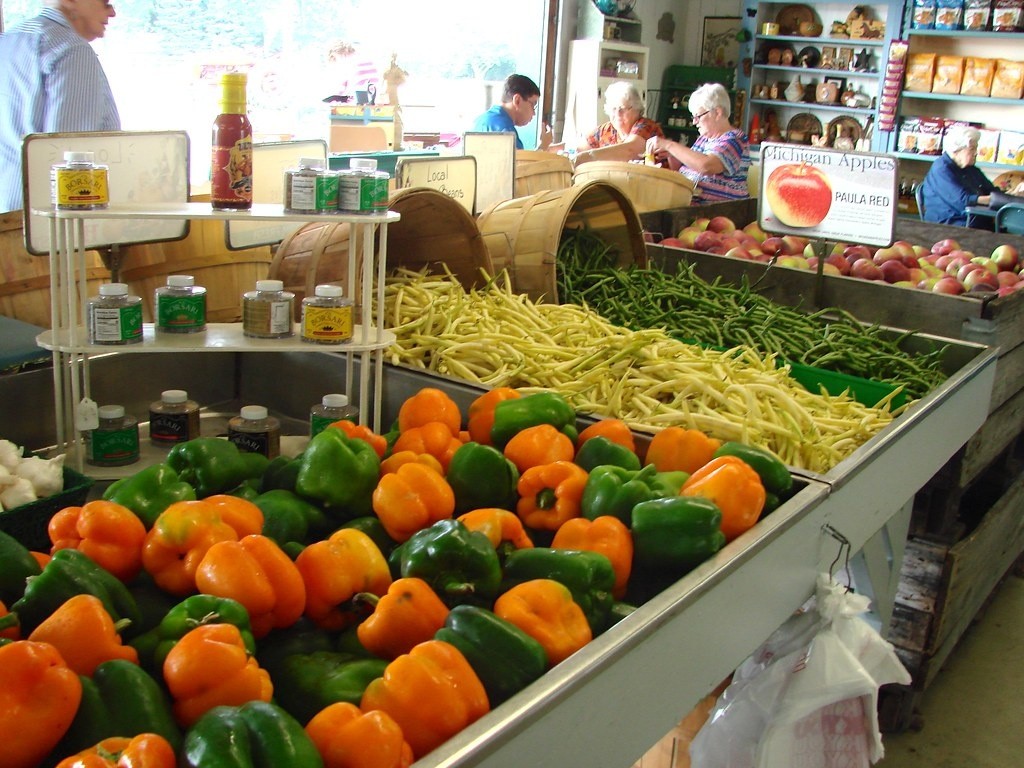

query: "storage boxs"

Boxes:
[0, 448, 95, 552]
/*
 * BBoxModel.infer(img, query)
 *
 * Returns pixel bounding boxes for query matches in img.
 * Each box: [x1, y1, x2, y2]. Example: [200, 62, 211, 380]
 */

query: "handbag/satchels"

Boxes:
[989, 192, 1024, 210]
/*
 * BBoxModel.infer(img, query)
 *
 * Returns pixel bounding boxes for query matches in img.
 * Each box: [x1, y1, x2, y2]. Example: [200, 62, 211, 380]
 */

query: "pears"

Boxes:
[641, 216, 1024, 298]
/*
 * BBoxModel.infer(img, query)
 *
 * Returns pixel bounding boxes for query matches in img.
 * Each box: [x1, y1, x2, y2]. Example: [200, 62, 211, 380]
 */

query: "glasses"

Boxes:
[607, 106, 633, 113]
[520, 96, 537, 111]
[691, 108, 713, 122]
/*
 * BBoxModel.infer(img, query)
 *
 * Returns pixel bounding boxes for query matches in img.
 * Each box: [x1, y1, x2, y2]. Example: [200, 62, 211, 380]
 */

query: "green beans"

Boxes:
[368, 225, 952, 475]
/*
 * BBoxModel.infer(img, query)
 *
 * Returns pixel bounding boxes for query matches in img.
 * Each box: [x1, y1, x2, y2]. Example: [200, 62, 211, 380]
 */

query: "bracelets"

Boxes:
[589, 150, 595, 161]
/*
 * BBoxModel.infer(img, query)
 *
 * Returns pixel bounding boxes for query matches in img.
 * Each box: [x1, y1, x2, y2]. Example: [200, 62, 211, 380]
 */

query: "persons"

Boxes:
[574, 82, 666, 165]
[473, 74, 564, 153]
[0, 0, 120, 213]
[328, 42, 376, 102]
[646, 82, 750, 206]
[922, 125, 1024, 232]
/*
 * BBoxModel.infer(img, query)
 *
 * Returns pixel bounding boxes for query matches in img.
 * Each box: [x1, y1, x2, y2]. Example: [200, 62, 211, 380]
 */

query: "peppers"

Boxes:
[0, 500, 184, 768]
[106, 387, 793, 768]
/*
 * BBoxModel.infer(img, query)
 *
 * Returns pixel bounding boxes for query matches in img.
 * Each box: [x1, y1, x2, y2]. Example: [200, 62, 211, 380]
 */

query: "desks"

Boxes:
[965, 206, 997, 230]
[0, 199, 1024, 767]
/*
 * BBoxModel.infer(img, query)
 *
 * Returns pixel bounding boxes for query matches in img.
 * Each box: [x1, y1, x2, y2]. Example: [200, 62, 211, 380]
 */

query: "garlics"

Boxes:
[0, 438, 68, 512]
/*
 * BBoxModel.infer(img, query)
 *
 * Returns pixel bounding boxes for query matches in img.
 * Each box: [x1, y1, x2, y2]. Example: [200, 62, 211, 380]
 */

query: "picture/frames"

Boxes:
[701, 16, 742, 67]
[825, 77, 847, 102]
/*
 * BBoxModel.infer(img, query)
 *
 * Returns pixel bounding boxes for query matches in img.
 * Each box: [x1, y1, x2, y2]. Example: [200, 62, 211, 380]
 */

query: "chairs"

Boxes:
[915, 181, 925, 221]
[995, 203, 1024, 235]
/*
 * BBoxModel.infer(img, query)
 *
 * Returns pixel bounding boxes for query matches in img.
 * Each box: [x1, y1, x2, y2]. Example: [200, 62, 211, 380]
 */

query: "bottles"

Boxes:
[51, 152, 110, 211]
[301, 285, 355, 344]
[243, 280, 295, 338]
[211, 73, 253, 209]
[673, 99, 678, 109]
[310, 394, 358, 440]
[283, 158, 339, 215]
[668, 114, 687, 127]
[899, 177, 924, 197]
[155, 275, 207, 334]
[568, 150, 576, 162]
[87, 283, 143, 345]
[228, 405, 280, 461]
[149, 390, 200, 447]
[85, 405, 140, 467]
[339, 158, 390, 215]
[762, 18, 779, 35]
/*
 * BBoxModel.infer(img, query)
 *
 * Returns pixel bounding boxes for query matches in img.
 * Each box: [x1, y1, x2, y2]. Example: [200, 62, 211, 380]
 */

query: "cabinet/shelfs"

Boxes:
[563, 0, 1024, 220]
[32, 203, 400, 480]
